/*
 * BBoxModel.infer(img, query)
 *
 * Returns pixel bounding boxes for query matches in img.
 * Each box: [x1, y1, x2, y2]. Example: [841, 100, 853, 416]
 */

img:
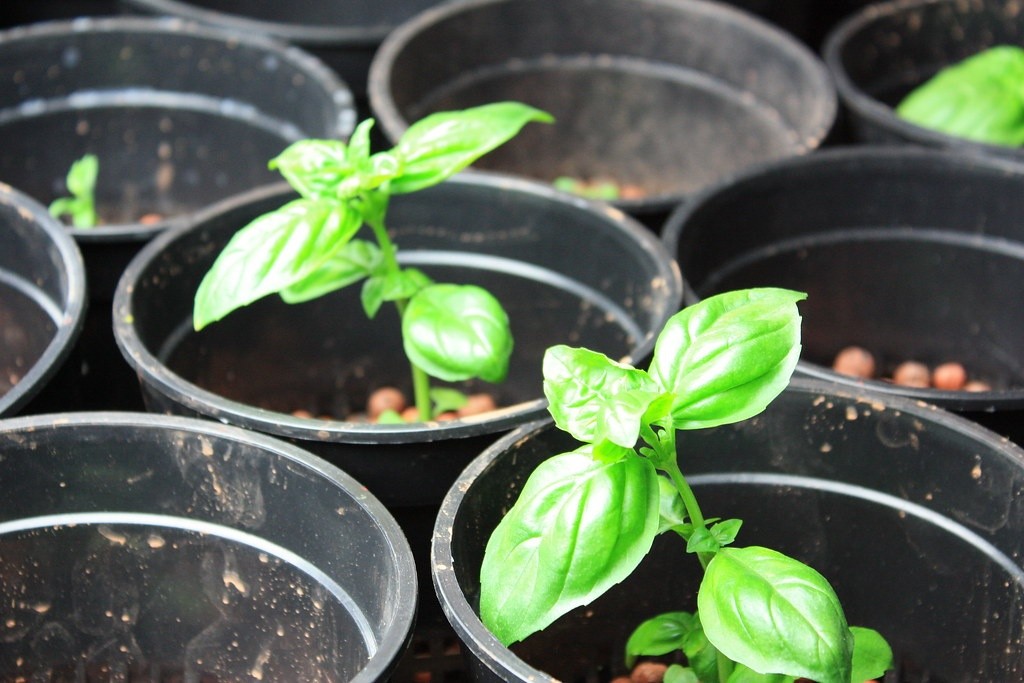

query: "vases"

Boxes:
[0, 410, 417, 683]
[664, 147, 1024, 446]
[0, 180, 85, 415]
[143, 0, 467, 140]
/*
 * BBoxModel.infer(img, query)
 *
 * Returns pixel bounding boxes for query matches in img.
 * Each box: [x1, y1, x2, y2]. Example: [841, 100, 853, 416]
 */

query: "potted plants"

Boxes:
[426, 286, 1024, 683]
[114, 100, 686, 661]
[364, 0, 836, 236]
[825, 0, 1024, 156]
[0, 18, 356, 409]
